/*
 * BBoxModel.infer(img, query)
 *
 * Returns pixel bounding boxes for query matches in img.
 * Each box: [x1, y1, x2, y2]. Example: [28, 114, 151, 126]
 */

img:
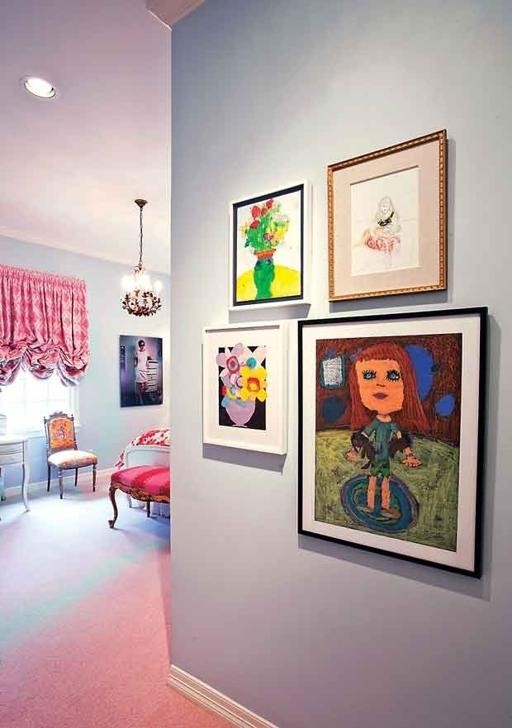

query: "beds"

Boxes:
[122, 427, 170, 509]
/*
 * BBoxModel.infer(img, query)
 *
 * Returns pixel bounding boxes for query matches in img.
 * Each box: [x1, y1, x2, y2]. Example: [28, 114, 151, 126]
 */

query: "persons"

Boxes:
[134, 339, 151, 404]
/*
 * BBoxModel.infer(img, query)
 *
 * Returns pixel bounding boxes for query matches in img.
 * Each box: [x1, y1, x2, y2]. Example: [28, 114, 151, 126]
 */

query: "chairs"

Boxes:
[43, 412, 98, 499]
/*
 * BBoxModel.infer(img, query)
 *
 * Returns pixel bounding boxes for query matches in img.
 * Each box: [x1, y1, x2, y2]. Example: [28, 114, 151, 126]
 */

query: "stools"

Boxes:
[108, 464, 170, 529]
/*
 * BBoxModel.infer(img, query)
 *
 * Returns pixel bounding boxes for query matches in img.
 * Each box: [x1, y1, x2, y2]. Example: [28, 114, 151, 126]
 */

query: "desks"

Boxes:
[0, 435, 31, 512]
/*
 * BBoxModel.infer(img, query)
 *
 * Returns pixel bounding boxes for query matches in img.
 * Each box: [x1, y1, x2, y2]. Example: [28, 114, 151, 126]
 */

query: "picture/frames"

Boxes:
[327, 129, 448, 303]
[298, 304, 490, 582]
[203, 319, 290, 457]
[227, 177, 314, 316]
